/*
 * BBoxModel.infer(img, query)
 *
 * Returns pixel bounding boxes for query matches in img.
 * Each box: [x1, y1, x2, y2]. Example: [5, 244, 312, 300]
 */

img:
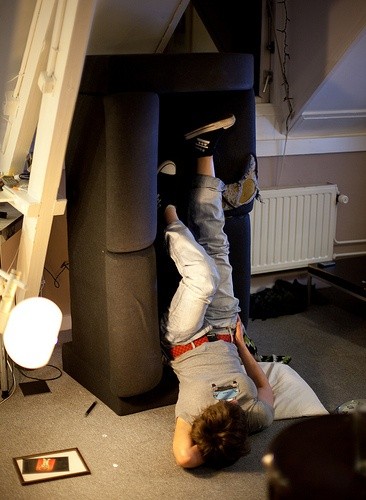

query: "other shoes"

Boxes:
[158, 160, 177, 214]
[184, 113, 236, 157]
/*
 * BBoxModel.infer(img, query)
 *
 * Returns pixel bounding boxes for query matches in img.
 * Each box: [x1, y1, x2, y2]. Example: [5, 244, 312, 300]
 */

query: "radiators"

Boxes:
[249, 182, 349, 275]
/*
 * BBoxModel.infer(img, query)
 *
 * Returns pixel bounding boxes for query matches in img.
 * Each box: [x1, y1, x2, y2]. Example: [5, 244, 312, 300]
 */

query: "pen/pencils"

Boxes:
[83, 400, 97, 418]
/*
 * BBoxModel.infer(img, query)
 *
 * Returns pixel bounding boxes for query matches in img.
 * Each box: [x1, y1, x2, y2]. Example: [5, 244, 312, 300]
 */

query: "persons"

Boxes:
[156, 113, 273, 468]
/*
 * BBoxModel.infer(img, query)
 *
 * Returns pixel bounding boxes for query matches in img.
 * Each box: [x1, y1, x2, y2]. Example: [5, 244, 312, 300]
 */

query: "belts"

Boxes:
[168, 333, 236, 361]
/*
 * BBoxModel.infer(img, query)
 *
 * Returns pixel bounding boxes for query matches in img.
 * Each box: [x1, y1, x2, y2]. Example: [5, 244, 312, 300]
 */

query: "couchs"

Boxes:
[62, 53, 255, 416]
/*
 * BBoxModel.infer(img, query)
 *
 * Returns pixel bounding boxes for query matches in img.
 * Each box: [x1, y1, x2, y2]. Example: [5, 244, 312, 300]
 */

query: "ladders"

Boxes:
[0, 0, 101, 398]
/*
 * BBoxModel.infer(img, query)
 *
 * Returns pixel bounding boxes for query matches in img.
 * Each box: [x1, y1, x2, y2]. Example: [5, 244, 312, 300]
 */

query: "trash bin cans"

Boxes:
[267, 412, 366, 500]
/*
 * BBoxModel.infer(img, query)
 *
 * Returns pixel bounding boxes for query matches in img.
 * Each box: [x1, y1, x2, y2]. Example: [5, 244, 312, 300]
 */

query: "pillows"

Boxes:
[241, 362, 330, 420]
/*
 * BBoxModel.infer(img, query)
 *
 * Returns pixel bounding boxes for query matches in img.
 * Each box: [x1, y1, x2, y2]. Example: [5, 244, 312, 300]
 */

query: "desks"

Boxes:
[307, 254, 366, 305]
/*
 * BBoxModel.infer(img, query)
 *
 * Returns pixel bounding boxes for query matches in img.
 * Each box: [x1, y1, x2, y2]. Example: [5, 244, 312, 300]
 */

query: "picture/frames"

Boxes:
[12, 447, 92, 486]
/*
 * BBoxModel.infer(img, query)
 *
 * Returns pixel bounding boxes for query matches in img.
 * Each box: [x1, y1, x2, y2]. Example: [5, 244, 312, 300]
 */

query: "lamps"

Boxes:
[0, 269, 63, 369]
